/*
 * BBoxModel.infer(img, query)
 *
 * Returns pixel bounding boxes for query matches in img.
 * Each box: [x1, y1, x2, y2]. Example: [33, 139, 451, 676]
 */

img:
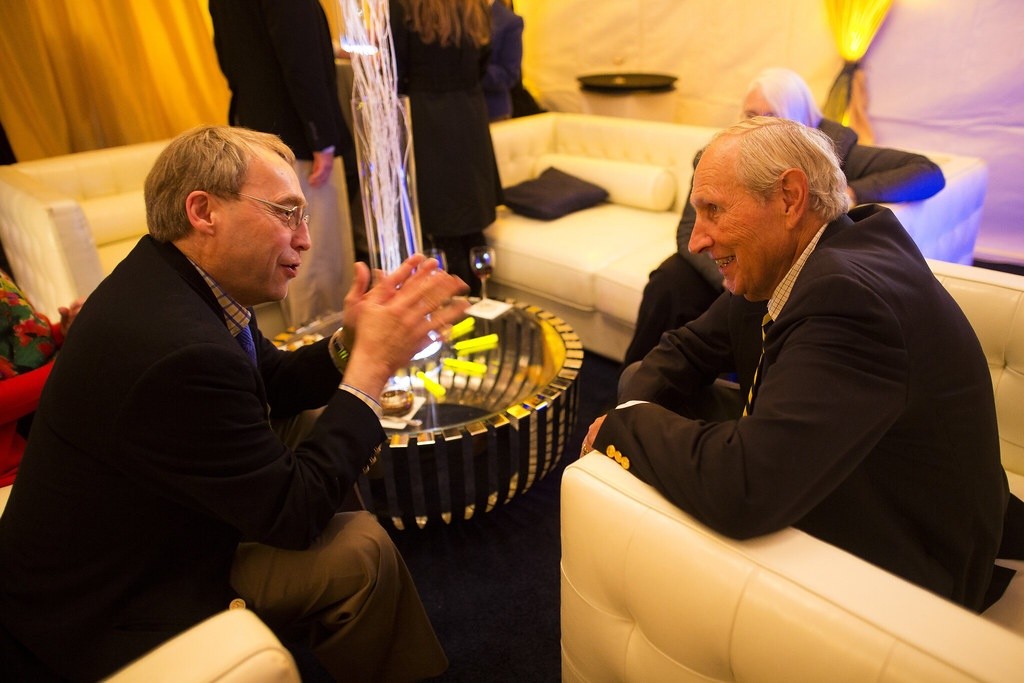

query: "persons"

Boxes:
[0, 271, 85, 489]
[580, 117, 1010, 613]
[208, 0, 353, 328]
[0, 125, 469, 683]
[377, 0, 525, 291]
[623, 68, 945, 369]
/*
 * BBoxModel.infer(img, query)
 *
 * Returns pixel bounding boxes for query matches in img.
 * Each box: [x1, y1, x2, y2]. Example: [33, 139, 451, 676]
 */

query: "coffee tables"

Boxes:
[273, 296, 584, 536]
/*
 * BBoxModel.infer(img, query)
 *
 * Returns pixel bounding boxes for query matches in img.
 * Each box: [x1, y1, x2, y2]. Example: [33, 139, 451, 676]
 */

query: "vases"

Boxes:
[352, 95, 424, 280]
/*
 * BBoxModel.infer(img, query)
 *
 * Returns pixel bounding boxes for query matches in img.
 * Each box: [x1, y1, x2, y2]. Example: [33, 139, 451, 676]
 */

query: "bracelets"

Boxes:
[334, 328, 349, 362]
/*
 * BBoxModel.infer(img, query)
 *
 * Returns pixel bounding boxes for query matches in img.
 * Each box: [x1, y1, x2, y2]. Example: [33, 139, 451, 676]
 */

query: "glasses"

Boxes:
[223, 189, 312, 232]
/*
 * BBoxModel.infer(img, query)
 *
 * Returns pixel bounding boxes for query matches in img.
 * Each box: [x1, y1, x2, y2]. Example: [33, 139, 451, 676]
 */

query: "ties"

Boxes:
[235, 314, 259, 372]
[739, 309, 774, 420]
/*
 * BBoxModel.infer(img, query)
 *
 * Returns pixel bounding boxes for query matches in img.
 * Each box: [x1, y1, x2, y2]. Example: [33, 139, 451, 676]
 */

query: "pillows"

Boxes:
[535, 153, 675, 211]
[501, 167, 608, 220]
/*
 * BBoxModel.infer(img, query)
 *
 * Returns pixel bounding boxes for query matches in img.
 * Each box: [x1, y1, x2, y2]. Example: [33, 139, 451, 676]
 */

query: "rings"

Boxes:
[583, 444, 589, 455]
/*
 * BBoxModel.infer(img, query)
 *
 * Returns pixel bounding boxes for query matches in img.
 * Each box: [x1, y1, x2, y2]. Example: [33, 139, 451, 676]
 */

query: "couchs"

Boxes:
[0, 109, 1024, 683]
